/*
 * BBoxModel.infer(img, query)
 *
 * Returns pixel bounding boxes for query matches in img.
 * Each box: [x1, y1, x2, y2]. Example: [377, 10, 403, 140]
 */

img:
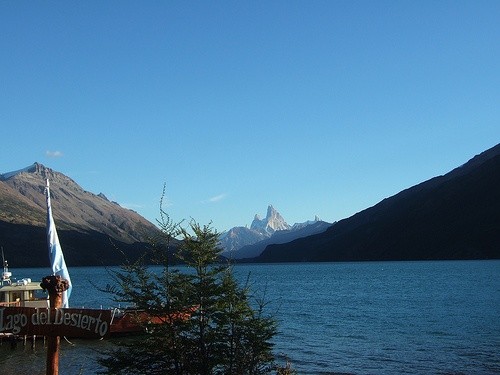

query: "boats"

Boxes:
[0, 251, 200, 336]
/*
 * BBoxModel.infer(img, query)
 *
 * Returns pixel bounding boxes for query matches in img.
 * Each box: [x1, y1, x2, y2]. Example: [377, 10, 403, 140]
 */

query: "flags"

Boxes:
[46, 190, 73, 308]
